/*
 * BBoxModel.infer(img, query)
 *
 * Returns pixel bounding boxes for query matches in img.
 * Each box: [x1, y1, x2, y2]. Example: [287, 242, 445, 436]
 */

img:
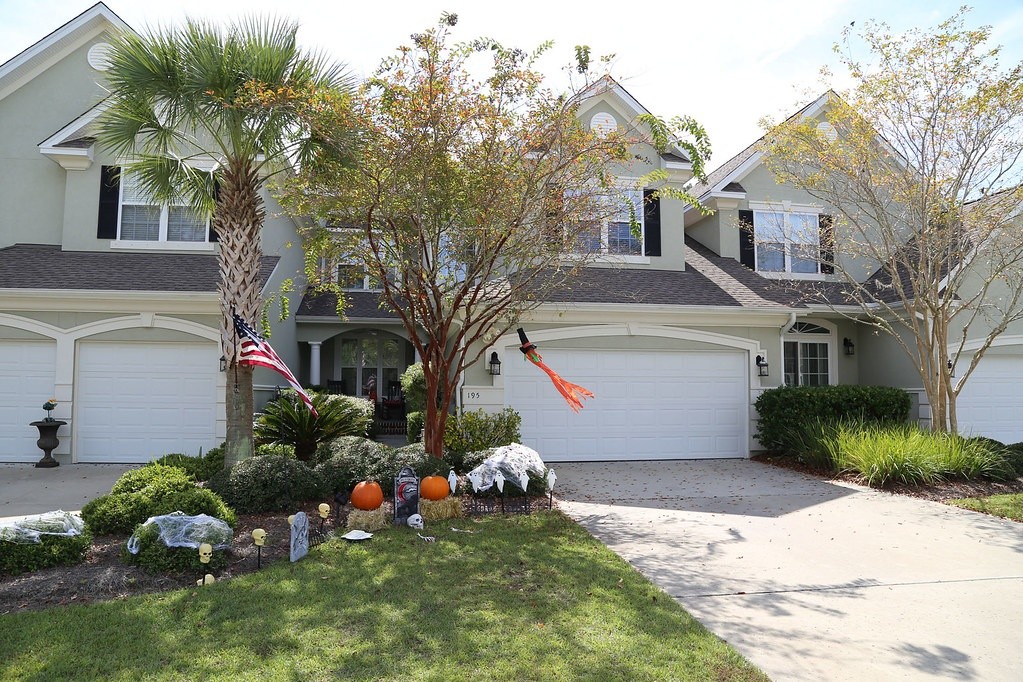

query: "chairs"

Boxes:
[381, 380, 405, 421]
[326, 379, 347, 395]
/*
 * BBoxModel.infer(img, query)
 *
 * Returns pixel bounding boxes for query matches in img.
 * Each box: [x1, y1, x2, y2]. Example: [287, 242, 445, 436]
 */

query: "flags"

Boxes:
[233, 314, 319, 418]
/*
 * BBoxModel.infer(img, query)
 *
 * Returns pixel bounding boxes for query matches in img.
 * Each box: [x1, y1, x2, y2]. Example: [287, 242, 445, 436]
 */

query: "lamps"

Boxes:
[755, 355, 769, 376]
[843, 337, 855, 355]
[218, 355, 225, 372]
[489, 351, 501, 376]
[948, 358, 955, 378]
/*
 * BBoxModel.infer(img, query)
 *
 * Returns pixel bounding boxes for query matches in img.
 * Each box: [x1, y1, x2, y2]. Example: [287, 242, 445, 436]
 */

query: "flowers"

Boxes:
[42, 398, 59, 423]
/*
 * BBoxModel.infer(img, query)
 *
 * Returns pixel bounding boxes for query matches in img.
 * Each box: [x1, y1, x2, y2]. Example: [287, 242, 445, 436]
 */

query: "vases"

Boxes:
[29, 421, 67, 468]
